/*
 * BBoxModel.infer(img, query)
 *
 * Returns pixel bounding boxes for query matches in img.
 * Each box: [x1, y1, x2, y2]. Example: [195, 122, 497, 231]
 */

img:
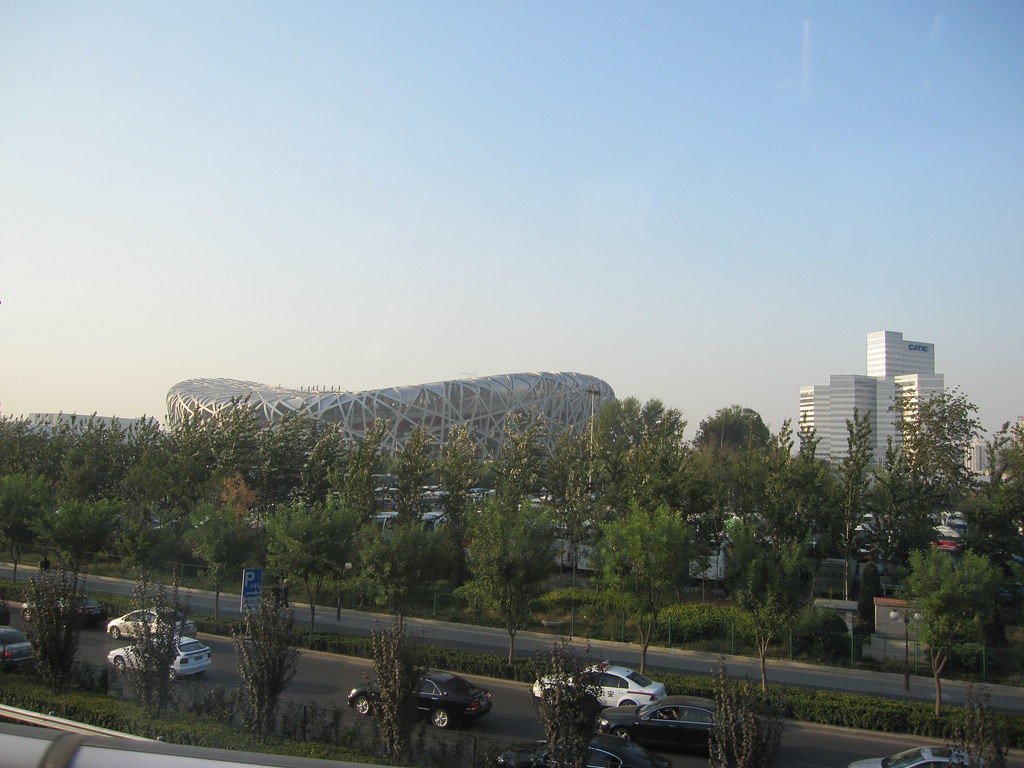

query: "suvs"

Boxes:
[107, 636, 212, 680]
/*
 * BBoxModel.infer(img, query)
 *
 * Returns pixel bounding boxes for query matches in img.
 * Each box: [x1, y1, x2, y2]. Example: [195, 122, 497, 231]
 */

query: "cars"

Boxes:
[0, 600, 10, 625]
[598, 695, 729, 748]
[0, 625, 33, 661]
[497, 733, 671, 768]
[21, 593, 107, 626]
[107, 607, 197, 641]
[533, 659, 667, 707]
[847, 745, 985, 768]
[346, 671, 493, 728]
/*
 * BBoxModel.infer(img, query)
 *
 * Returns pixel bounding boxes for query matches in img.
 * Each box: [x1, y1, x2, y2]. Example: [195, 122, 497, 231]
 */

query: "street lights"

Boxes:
[337, 562, 352, 621]
[890, 607, 925, 690]
[585, 389, 601, 510]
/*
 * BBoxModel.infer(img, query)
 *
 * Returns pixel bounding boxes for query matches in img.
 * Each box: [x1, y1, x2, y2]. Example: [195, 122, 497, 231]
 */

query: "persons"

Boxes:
[658, 709, 680, 720]
[272, 579, 289, 609]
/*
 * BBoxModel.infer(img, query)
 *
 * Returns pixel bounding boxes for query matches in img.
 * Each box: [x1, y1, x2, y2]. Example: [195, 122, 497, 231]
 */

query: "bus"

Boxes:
[536, 495, 602, 571]
[812, 515, 876, 592]
[690, 534, 752, 581]
[988, 556, 1024, 601]
[931, 510, 968, 553]
[372, 484, 495, 531]
